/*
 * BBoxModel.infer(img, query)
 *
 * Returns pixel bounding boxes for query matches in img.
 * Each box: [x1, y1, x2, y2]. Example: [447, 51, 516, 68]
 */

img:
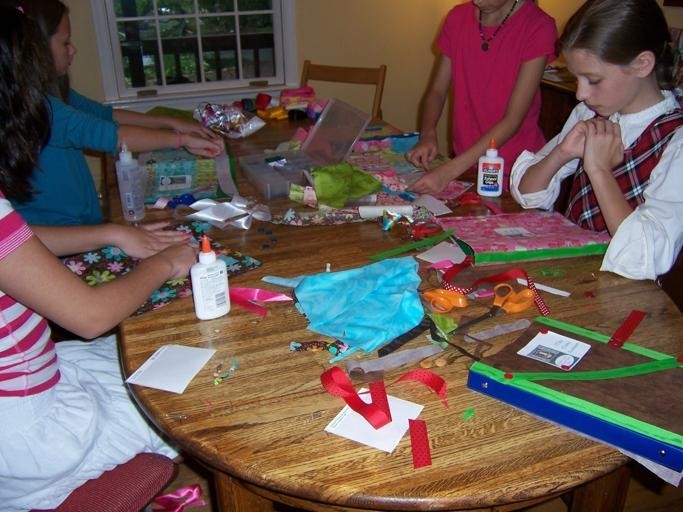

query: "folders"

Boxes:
[436, 212, 612, 269]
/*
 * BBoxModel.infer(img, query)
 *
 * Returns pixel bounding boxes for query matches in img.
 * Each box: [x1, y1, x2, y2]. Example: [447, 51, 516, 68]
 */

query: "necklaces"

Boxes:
[479, 1, 517, 51]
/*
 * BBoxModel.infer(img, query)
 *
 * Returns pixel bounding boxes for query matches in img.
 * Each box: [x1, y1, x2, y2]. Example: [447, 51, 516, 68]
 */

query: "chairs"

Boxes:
[297, 56, 387, 120]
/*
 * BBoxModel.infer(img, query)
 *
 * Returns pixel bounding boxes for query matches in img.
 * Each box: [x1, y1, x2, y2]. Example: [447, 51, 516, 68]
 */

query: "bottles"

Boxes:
[114, 144, 148, 220]
[475, 138, 503, 198]
[191, 235, 232, 321]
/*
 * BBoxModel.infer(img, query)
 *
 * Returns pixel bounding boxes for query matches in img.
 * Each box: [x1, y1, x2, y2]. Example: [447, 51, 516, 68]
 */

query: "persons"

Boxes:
[510, 1, 682, 288]
[1, 1, 223, 262]
[404, 0, 559, 196]
[0, 0, 199, 510]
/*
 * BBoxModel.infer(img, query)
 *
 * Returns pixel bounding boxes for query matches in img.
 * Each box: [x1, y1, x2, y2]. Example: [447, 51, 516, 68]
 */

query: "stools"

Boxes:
[33, 451, 175, 511]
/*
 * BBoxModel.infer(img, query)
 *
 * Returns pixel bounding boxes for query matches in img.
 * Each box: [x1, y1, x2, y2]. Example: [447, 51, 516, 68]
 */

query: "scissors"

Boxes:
[449, 283, 536, 335]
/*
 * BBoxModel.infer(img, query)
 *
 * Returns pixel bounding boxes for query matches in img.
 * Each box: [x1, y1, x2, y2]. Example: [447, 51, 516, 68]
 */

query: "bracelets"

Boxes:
[177, 131, 184, 149]
[449, 156, 474, 174]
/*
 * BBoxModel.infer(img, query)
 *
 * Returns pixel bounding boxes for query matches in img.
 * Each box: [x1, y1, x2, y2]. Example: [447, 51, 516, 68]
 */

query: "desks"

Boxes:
[537, 68, 583, 141]
[99, 108, 683, 512]
